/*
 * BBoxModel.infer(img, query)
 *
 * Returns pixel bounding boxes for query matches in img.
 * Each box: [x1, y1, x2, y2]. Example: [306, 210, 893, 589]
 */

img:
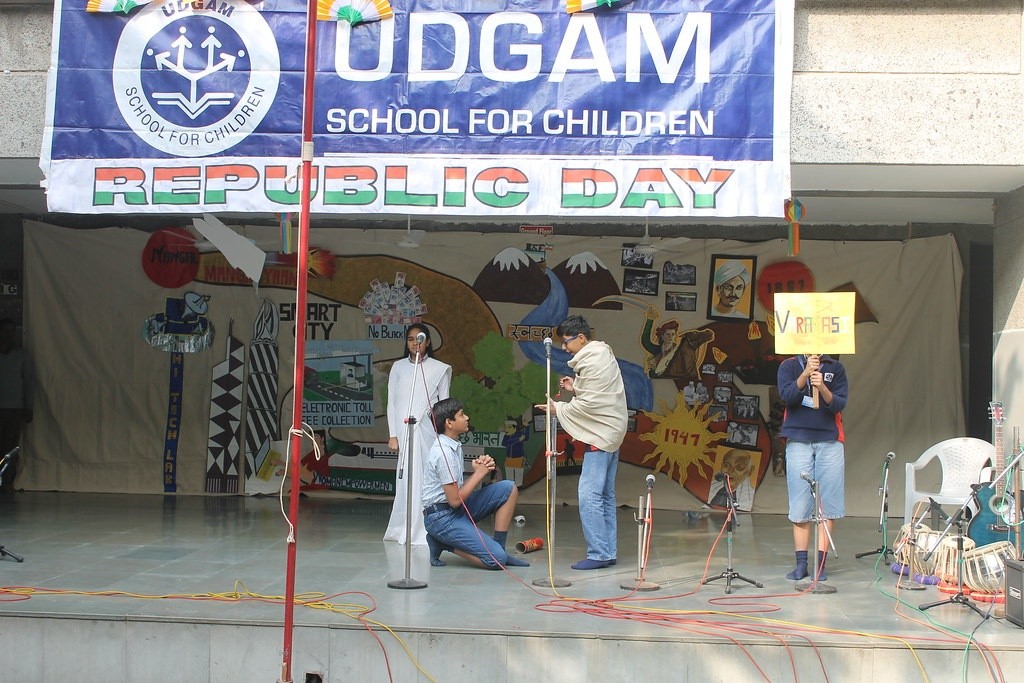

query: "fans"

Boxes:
[571, 217, 691, 254]
[348, 214, 453, 249]
[161, 215, 279, 252]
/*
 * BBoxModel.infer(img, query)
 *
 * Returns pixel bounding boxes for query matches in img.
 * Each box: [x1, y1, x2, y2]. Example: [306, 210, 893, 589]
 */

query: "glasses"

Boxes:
[561, 334, 586, 344]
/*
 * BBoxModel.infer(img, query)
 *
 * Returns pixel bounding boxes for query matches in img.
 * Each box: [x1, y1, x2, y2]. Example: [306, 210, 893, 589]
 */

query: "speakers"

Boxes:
[1004, 559, 1024, 628]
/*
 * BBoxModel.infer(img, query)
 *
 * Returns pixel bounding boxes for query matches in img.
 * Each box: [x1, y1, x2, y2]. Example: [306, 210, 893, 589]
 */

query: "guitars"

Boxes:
[966, 400, 1024, 549]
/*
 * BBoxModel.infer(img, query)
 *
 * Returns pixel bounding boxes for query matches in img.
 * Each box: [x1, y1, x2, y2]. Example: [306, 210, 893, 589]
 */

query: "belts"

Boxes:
[423, 503, 450, 516]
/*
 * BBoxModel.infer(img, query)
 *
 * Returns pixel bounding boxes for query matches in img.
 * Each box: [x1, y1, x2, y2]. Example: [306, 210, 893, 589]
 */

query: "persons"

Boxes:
[777, 354, 848, 581]
[535, 315, 629, 570]
[421, 398, 531, 570]
[383, 323, 453, 545]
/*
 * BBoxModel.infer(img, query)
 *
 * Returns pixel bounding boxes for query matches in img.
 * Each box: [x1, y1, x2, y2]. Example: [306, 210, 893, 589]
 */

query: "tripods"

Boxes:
[919, 484, 990, 619]
[701, 477, 763, 594]
[855, 457, 894, 565]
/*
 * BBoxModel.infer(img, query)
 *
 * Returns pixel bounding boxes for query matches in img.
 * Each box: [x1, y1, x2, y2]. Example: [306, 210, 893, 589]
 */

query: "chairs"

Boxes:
[904, 437, 998, 532]
[0, 405, 33, 563]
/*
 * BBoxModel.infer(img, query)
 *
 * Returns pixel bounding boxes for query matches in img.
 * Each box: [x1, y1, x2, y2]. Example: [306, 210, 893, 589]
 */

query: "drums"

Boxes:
[893, 523, 1017, 595]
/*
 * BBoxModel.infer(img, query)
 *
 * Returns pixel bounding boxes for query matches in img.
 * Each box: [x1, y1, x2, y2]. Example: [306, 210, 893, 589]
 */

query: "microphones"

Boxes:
[3, 446, 20, 460]
[929, 497, 952, 524]
[543, 337, 553, 359]
[646, 474, 656, 493]
[800, 471, 816, 486]
[715, 473, 728, 481]
[886, 451, 895, 462]
[417, 332, 426, 344]
